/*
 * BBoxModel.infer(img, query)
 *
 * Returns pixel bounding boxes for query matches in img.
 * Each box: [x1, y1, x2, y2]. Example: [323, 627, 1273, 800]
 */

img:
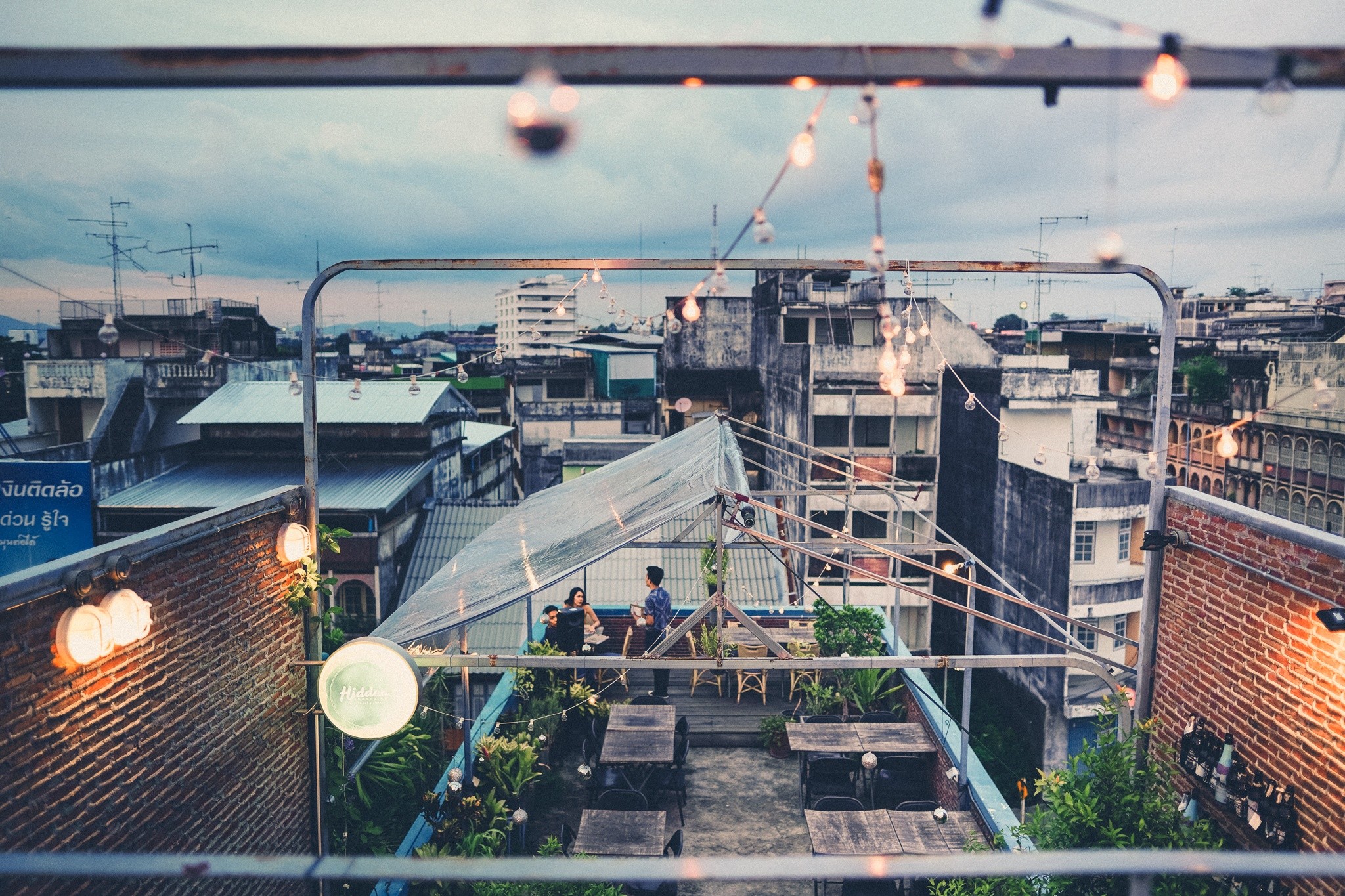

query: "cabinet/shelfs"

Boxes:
[1172, 737, 1299, 896]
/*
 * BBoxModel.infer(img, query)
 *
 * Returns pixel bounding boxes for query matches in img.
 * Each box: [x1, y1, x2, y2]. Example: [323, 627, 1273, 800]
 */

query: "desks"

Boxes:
[888, 809, 995, 896]
[854, 722, 938, 809]
[607, 704, 677, 776]
[599, 731, 674, 791]
[785, 721, 865, 816]
[582, 633, 610, 682]
[803, 809, 905, 896]
[571, 809, 667, 856]
[719, 627, 887, 698]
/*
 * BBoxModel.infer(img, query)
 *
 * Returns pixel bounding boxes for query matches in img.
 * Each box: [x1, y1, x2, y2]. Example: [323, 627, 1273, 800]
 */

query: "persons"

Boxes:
[564, 587, 601, 633]
[631, 566, 672, 699]
[543, 605, 601, 700]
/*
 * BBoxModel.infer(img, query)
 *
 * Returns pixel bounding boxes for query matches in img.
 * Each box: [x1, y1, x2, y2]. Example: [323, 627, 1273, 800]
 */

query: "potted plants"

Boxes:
[698, 534, 732, 625]
[756, 712, 799, 759]
[780, 599, 907, 723]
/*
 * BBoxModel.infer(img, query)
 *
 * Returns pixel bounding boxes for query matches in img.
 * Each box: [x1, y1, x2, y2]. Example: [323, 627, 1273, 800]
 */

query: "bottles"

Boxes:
[1179, 715, 1297, 896]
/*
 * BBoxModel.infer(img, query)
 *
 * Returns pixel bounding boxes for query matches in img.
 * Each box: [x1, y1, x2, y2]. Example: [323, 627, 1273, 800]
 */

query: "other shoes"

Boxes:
[648, 689, 669, 699]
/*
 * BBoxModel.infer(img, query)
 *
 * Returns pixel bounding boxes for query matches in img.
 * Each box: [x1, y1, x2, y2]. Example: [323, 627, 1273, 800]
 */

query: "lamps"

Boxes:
[278, 496, 313, 564]
[99, 554, 154, 646]
[56, 570, 112, 666]
[1315, 608, 1345, 632]
[1139, 528, 1190, 552]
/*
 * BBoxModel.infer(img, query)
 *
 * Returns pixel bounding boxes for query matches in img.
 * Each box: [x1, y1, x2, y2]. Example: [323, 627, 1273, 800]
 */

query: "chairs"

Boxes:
[686, 620, 821, 707]
[804, 711, 924, 812]
[625, 828, 684, 896]
[597, 789, 649, 810]
[581, 695, 689, 827]
[814, 799, 984, 896]
[564, 627, 633, 694]
[561, 825, 578, 859]
